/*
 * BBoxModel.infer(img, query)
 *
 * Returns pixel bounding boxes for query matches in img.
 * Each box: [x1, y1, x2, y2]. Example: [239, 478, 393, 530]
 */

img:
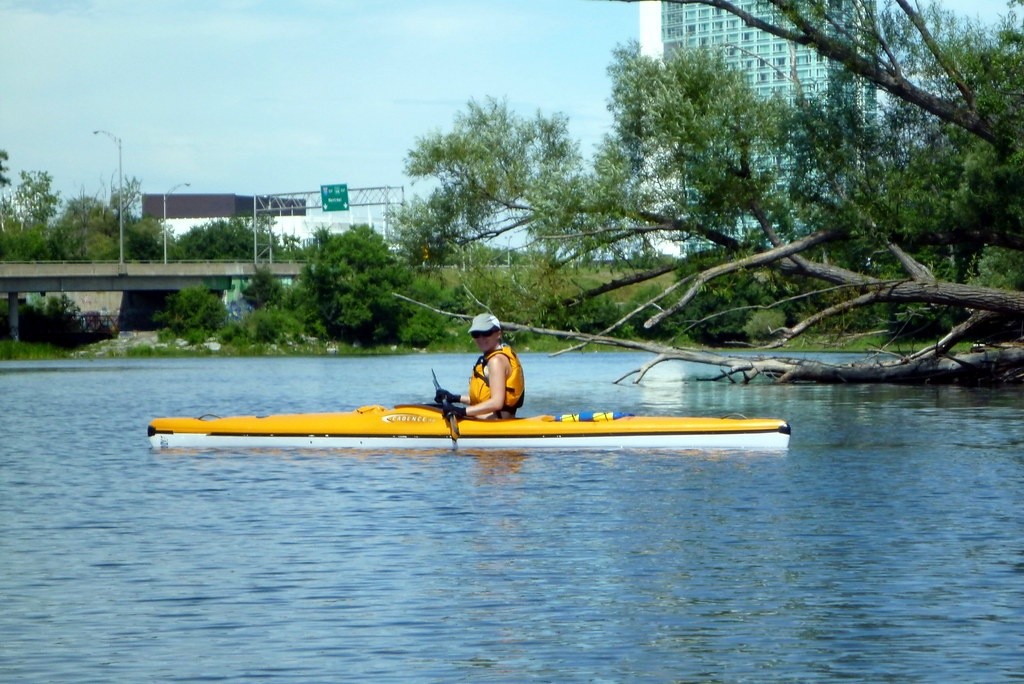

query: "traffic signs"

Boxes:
[321, 183, 349, 212]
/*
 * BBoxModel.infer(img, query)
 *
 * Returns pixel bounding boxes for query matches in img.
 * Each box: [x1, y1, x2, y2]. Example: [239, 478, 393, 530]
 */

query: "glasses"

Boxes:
[471, 330, 496, 338]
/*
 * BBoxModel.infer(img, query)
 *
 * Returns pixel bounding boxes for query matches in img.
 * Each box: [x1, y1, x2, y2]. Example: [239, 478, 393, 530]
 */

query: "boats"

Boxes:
[147, 402, 793, 450]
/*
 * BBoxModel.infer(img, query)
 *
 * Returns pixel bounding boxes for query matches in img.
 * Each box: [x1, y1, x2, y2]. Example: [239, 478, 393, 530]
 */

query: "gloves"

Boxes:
[443, 404, 467, 419]
[434, 389, 461, 403]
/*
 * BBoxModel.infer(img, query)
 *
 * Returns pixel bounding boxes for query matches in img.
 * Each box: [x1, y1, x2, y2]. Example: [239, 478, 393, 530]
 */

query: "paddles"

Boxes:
[431, 367, 460, 451]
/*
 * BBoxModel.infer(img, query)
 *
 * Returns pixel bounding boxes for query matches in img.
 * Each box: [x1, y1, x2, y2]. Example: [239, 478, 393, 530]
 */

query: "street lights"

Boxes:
[163, 182, 192, 264]
[93, 128, 124, 263]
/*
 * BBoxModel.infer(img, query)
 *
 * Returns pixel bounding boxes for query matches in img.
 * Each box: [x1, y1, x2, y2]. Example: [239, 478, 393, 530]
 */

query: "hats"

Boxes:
[467, 313, 500, 333]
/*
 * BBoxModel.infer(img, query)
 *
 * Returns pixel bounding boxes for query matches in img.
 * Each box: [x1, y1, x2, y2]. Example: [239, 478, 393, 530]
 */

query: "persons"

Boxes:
[430, 314, 525, 421]
